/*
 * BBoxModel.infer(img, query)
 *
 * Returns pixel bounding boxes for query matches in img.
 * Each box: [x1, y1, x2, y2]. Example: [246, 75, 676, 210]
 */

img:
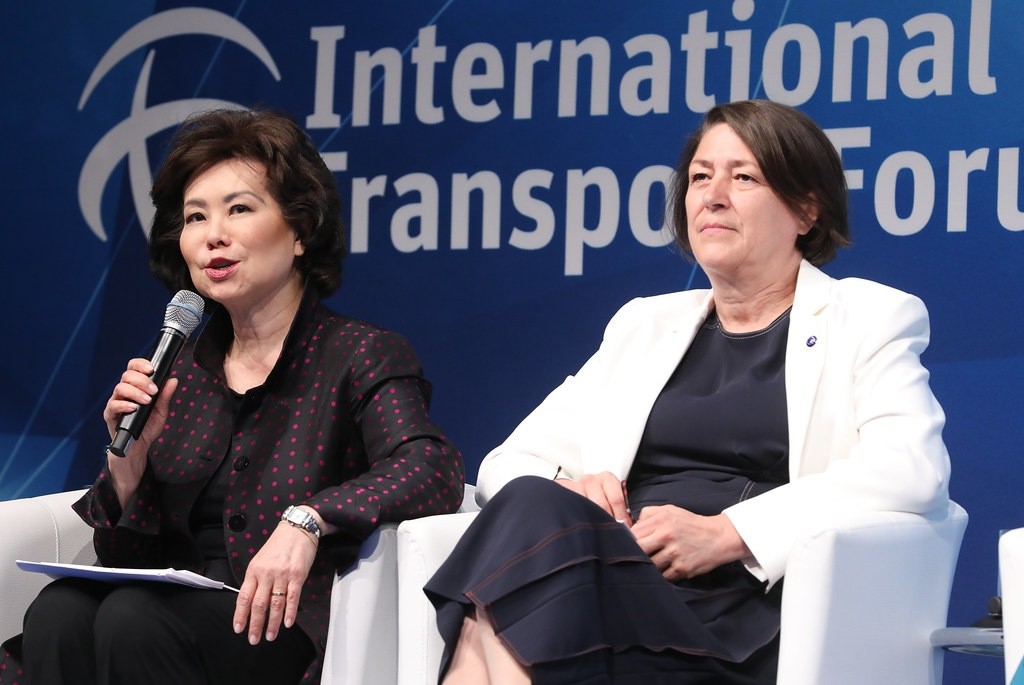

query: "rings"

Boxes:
[271, 592, 286, 597]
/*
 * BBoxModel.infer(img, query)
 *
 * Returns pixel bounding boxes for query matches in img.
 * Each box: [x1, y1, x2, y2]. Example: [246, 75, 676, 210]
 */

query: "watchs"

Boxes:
[281, 505, 322, 539]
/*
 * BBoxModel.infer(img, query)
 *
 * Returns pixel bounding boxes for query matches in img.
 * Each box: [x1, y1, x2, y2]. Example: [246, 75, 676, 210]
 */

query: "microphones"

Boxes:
[110, 290, 205, 458]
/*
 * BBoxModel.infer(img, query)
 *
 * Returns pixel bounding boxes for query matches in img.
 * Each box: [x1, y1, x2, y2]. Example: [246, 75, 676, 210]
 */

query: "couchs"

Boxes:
[0, 482, 482, 685]
[395, 500, 970, 685]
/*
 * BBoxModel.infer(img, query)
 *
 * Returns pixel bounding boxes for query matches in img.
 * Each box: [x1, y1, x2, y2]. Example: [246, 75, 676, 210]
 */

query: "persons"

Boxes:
[23, 110, 466, 685]
[421, 101, 951, 685]
